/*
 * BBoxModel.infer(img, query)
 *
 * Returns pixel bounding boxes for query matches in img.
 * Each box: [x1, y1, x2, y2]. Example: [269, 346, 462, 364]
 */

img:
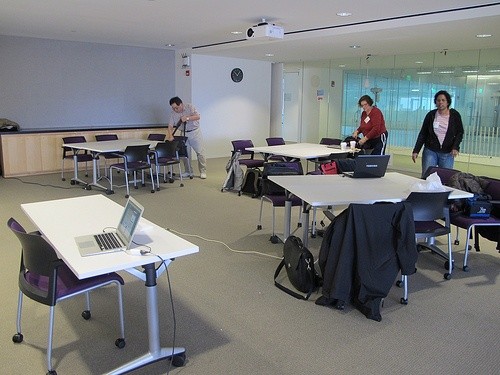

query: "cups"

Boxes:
[340, 142, 347, 151]
[350, 141, 356, 150]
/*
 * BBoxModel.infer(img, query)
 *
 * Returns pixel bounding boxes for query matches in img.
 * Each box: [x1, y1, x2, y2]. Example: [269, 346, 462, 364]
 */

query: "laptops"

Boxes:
[74, 195, 144, 257]
[343, 154, 390, 178]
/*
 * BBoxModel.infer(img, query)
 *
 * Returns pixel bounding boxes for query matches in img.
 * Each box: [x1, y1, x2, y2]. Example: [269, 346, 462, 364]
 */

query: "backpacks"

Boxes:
[238, 167, 263, 198]
[450, 171, 492, 218]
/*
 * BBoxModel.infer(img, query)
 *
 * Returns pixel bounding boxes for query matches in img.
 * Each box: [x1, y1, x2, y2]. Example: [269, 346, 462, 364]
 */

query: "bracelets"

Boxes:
[187, 117, 189, 121]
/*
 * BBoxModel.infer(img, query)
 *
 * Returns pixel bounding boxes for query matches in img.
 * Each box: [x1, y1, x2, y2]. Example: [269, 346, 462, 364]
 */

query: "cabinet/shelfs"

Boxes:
[0, 124, 181, 178]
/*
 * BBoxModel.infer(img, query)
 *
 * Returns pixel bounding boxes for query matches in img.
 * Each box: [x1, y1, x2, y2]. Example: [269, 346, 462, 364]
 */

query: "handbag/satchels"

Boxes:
[274, 235, 323, 301]
[166, 119, 189, 150]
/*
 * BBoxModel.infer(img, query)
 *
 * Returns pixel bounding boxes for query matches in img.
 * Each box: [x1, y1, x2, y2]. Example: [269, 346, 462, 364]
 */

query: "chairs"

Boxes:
[229, 136, 500, 307]
[6, 216, 126, 375]
[61, 133, 188, 198]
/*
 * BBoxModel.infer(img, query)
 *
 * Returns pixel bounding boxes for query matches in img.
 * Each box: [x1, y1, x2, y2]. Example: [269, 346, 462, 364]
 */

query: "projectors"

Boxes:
[246, 24, 285, 39]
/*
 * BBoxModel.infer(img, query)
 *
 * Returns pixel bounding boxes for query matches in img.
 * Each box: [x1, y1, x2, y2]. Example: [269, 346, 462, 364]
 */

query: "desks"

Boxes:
[267, 171, 474, 248]
[19, 193, 199, 375]
[62, 139, 174, 190]
[244, 143, 356, 175]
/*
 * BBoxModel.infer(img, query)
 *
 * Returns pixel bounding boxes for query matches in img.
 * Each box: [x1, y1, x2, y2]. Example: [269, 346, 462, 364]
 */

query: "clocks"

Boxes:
[231, 68, 243, 82]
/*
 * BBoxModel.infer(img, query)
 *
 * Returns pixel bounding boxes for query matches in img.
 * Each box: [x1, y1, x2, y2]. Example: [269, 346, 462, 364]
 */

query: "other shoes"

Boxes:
[200, 173, 206, 178]
[178, 174, 190, 179]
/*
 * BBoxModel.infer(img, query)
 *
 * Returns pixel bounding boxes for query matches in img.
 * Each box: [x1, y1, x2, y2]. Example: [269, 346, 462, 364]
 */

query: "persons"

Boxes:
[167, 96, 207, 179]
[412, 91, 466, 177]
[353, 95, 388, 155]
